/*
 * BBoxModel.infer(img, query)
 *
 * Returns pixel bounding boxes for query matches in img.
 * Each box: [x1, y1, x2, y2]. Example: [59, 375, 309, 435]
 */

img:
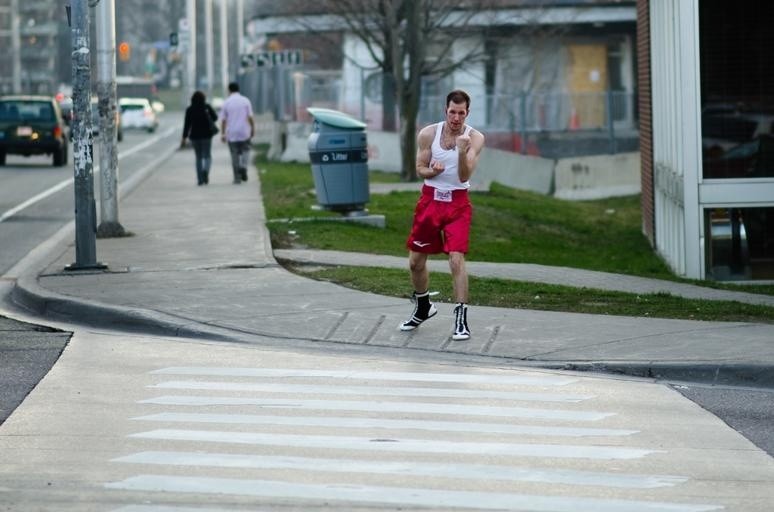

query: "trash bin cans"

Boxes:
[306, 107, 370, 212]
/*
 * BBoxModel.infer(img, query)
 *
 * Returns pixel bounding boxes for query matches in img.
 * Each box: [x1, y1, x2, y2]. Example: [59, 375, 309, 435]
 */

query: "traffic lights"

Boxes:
[119, 42, 129, 61]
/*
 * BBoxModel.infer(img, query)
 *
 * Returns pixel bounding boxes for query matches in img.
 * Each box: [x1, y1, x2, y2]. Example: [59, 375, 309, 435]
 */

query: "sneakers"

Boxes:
[232, 167, 248, 184]
[197, 170, 208, 185]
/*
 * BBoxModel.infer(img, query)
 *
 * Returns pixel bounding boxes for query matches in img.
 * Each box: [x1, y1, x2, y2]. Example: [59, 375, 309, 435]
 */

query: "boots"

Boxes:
[453, 302, 471, 340]
[400, 290, 438, 331]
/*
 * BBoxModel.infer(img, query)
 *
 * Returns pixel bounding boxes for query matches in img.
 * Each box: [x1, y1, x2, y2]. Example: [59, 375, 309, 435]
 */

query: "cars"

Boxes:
[0, 95, 158, 166]
[702, 94, 774, 178]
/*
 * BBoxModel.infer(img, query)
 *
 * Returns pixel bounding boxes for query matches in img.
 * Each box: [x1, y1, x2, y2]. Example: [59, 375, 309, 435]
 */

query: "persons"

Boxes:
[400, 91, 485, 340]
[183, 83, 255, 185]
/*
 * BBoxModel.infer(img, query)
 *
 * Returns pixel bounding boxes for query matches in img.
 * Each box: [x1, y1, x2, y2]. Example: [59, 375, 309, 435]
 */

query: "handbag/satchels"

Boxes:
[204, 104, 220, 135]
